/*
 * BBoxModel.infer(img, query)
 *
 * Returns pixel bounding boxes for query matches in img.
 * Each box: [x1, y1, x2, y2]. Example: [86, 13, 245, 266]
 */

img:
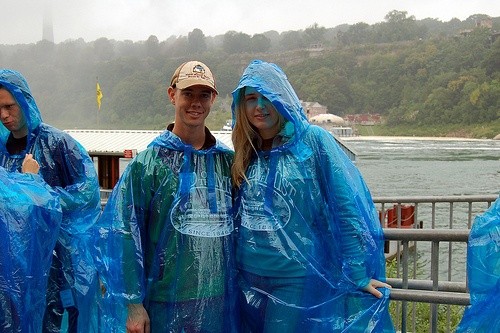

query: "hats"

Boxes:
[170, 61, 219, 96]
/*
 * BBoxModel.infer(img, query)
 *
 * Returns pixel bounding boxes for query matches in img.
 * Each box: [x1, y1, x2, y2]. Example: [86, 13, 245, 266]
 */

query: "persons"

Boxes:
[0, 68, 99, 333]
[230, 58, 392, 333]
[112, 60, 236, 332]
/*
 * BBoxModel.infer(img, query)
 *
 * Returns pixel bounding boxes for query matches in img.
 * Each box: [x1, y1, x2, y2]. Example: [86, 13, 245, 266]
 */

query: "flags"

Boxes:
[97, 83, 103, 110]
